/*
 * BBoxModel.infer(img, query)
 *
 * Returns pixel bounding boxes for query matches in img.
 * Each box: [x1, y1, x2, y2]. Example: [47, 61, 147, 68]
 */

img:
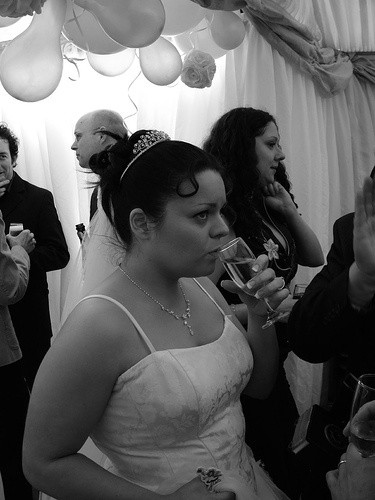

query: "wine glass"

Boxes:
[217, 238, 289, 328]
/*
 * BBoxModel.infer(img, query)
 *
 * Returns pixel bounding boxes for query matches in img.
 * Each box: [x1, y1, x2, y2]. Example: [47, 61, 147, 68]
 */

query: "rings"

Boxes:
[275, 277, 287, 290]
[337, 459, 345, 466]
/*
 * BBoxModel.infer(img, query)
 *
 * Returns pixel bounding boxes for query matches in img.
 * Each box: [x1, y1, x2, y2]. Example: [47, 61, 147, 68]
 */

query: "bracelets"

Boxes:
[229, 302, 236, 313]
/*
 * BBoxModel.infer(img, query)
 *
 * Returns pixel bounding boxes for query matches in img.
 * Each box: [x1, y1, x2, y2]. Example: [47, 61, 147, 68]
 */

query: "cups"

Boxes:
[9, 223, 23, 246]
[293, 284, 307, 299]
[350, 374, 375, 457]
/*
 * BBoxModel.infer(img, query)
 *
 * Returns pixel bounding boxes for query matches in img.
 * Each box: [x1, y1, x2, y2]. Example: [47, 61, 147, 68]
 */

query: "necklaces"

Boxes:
[117, 264, 199, 338]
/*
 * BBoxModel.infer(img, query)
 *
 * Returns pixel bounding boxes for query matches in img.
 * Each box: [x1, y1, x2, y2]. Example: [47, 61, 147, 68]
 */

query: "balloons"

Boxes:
[1, 1, 252, 102]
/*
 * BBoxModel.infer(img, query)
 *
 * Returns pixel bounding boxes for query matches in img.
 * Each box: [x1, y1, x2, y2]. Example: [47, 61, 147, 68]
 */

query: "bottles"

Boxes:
[76, 223, 89, 267]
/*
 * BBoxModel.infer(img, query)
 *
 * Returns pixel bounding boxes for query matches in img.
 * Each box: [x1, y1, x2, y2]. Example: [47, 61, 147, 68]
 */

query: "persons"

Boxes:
[0, 127, 72, 500]
[289, 164, 375, 499]
[203, 107, 325, 500]
[21, 130, 289, 499]
[57, 110, 130, 339]
[0, 209, 40, 500]
[322, 397, 375, 498]
